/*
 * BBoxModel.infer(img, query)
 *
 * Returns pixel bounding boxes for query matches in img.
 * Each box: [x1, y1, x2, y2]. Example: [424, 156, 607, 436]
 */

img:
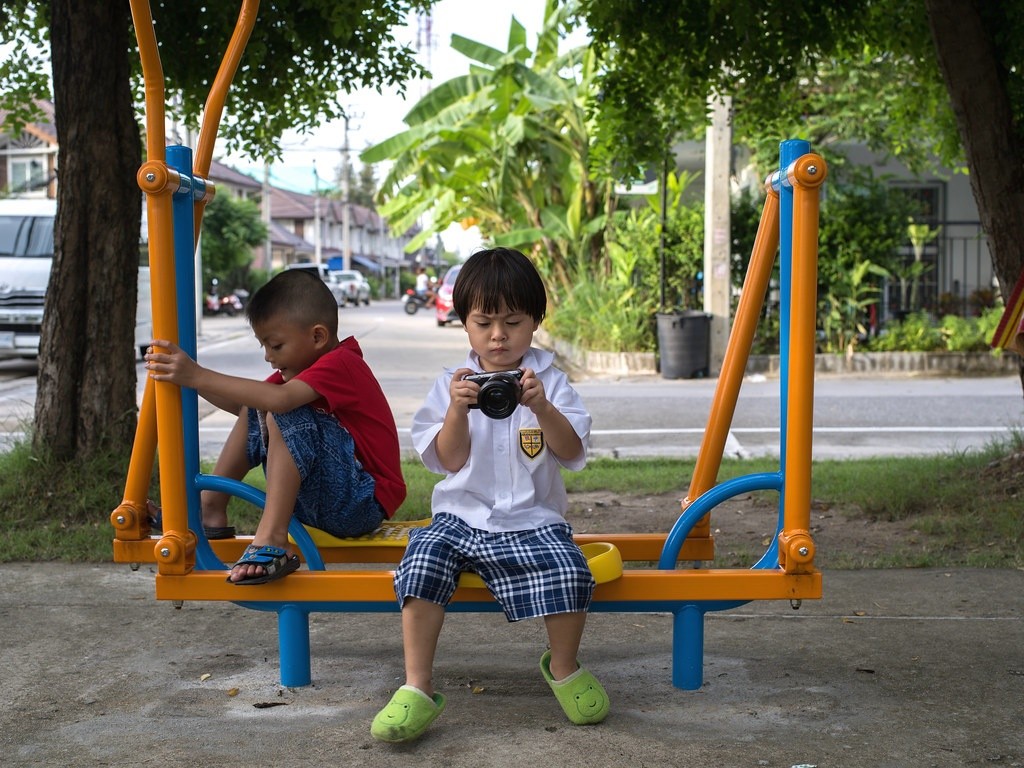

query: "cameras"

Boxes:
[463, 369, 523, 419]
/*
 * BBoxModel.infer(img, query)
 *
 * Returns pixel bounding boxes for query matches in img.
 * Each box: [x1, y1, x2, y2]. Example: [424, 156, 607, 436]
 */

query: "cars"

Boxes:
[333, 269, 373, 307]
[429, 260, 465, 327]
[285, 262, 347, 310]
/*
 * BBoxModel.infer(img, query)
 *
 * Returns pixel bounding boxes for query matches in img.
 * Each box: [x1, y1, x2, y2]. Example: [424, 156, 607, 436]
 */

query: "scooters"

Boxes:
[205, 277, 244, 316]
[401, 274, 441, 314]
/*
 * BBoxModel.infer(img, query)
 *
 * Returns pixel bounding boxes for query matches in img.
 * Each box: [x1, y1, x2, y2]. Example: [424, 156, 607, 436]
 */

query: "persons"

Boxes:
[369, 248, 611, 742]
[415, 268, 436, 309]
[147, 268, 407, 589]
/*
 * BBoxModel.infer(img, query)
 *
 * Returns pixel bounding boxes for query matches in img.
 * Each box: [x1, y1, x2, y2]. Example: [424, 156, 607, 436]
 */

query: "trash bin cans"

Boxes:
[655, 310, 714, 378]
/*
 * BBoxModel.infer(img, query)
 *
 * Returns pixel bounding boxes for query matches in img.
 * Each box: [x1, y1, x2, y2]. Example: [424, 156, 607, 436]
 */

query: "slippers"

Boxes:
[225, 544, 301, 584]
[540, 649, 610, 724]
[370, 685, 447, 742]
[147, 507, 236, 539]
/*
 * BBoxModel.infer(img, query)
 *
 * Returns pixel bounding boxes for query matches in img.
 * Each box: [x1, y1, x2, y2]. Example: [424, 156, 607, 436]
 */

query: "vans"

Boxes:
[0, 198, 205, 370]
